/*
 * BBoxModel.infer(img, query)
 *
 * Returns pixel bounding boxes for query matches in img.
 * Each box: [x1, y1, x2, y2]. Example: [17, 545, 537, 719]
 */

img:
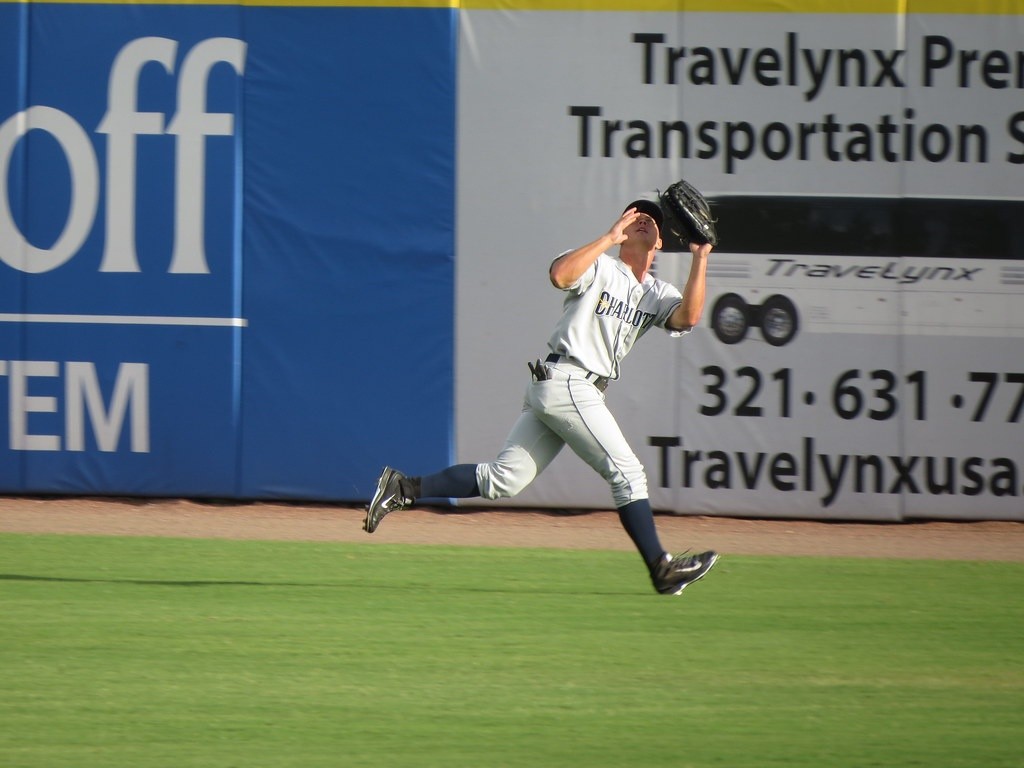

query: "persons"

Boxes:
[362, 178, 722, 596]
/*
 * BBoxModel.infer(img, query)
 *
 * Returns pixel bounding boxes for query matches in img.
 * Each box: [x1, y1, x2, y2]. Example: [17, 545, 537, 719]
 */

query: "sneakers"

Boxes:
[362, 465, 413, 533]
[651, 549, 721, 596]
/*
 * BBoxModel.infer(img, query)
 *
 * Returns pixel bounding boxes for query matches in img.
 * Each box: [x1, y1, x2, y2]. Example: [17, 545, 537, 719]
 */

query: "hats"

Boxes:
[620, 199, 664, 235]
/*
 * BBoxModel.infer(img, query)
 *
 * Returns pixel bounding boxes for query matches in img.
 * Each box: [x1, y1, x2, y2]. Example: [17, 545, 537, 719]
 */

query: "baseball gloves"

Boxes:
[657, 179, 719, 245]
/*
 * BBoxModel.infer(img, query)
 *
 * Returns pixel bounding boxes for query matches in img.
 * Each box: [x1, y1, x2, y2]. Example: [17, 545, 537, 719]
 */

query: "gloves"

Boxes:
[528, 359, 555, 383]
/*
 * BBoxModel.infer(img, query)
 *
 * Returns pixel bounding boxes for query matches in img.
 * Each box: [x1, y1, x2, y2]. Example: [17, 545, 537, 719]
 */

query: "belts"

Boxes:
[547, 353, 610, 393]
